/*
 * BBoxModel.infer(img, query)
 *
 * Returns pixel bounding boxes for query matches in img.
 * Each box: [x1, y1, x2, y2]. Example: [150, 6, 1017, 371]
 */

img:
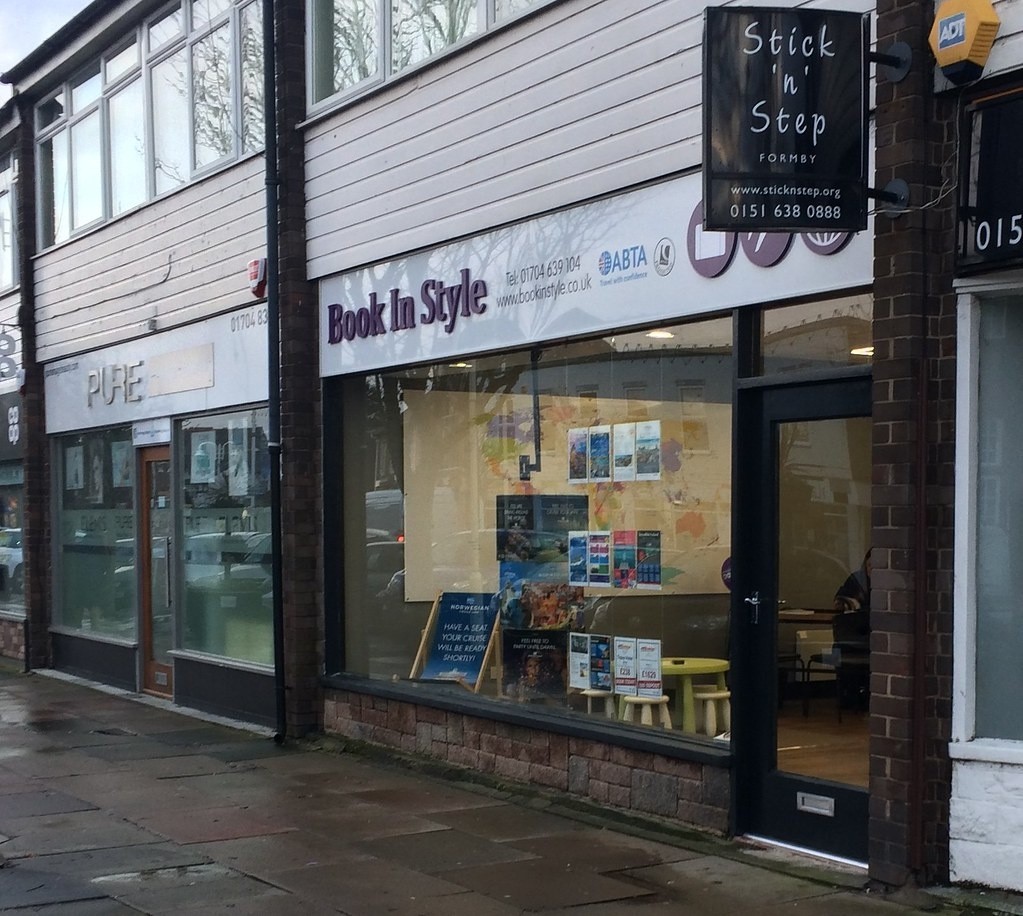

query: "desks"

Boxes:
[609, 657, 730, 734]
[777, 612, 838, 673]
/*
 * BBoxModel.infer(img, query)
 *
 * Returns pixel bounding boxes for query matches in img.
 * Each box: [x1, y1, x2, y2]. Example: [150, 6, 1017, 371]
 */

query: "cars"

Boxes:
[1, 526, 30, 593]
[71, 486, 861, 711]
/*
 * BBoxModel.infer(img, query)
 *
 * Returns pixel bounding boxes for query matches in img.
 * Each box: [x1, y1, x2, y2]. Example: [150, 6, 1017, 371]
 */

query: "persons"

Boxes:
[597, 659, 602, 669]
[572, 640, 586, 652]
[620, 661, 630, 676]
[642, 663, 656, 678]
[595, 644, 602, 657]
[506, 582, 583, 628]
[605, 661, 609, 670]
[601, 675, 608, 684]
[833, 547, 872, 710]
[507, 647, 567, 705]
[65, 521, 111, 632]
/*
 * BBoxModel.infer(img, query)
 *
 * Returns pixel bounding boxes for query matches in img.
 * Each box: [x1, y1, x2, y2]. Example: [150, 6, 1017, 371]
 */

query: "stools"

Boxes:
[692, 685, 731, 737]
[776, 650, 871, 725]
[584, 689, 673, 729]
